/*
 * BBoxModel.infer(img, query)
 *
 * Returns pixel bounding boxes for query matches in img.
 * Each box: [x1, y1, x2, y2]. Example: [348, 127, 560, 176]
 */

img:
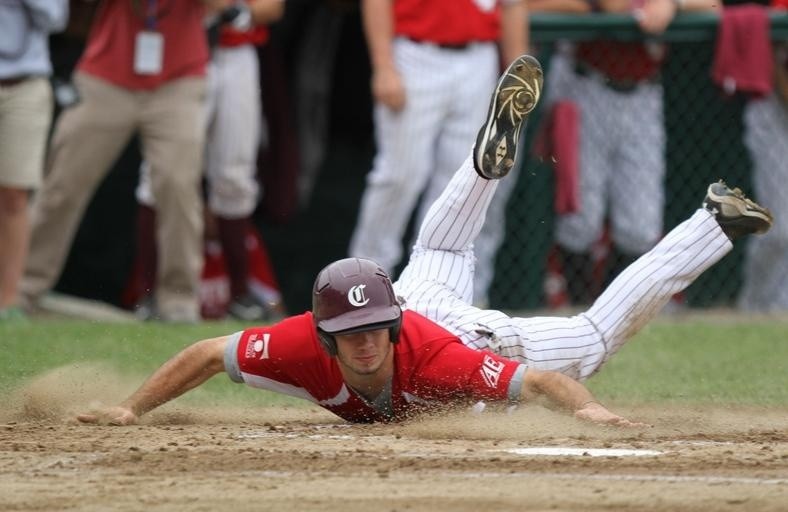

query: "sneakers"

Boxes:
[472, 55, 543, 178]
[703, 181, 775, 238]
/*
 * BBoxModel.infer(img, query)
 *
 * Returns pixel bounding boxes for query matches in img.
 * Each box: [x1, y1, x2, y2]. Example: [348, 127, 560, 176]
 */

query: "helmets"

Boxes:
[311, 256, 401, 355]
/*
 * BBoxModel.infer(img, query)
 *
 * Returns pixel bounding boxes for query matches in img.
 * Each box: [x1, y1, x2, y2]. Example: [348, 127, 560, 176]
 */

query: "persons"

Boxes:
[0, 0, 73, 312]
[70, 50, 775, 437]
